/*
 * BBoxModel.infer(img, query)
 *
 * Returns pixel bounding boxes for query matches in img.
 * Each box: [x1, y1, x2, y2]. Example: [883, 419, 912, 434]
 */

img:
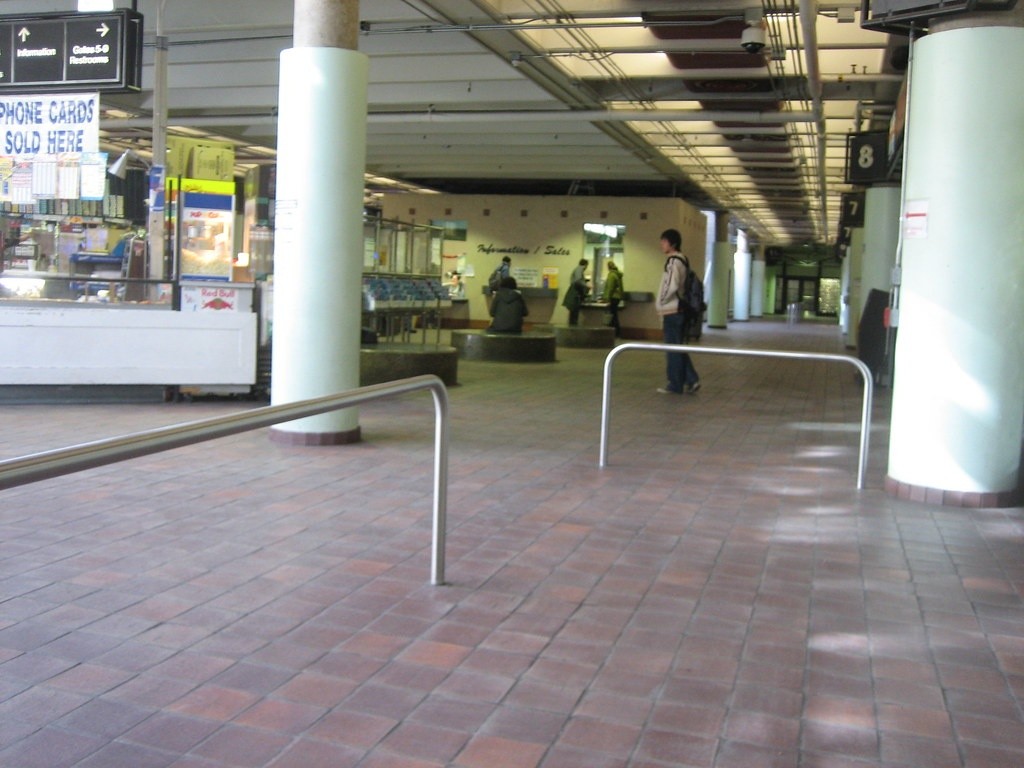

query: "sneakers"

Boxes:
[656, 384, 675, 394]
[686, 382, 701, 395]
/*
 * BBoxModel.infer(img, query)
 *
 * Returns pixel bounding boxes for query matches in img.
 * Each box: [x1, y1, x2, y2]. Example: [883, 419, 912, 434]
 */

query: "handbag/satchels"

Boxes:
[601, 307, 614, 324]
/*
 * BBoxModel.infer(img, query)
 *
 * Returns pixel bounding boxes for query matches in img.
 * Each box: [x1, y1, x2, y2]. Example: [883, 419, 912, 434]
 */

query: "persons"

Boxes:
[487, 277, 529, 335]
[489, 256, 511, 295]
[655, 229, 702, 396]
[569, 258, 591, 325]
[603, 261, 623, 338]
[449, 272, 465, 297]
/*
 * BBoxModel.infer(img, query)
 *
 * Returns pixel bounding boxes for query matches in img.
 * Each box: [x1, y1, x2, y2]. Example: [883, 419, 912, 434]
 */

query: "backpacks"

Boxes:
[663, 256, 707, 318]
[489, 264, 508, 291]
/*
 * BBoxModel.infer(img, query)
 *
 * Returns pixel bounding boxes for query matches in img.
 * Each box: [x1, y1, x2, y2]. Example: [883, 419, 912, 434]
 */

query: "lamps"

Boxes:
[108, 150, 151, 180]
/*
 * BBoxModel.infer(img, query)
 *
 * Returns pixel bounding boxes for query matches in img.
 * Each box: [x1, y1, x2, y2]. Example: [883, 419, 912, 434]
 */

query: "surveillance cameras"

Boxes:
[740, 28, 766, 53]
